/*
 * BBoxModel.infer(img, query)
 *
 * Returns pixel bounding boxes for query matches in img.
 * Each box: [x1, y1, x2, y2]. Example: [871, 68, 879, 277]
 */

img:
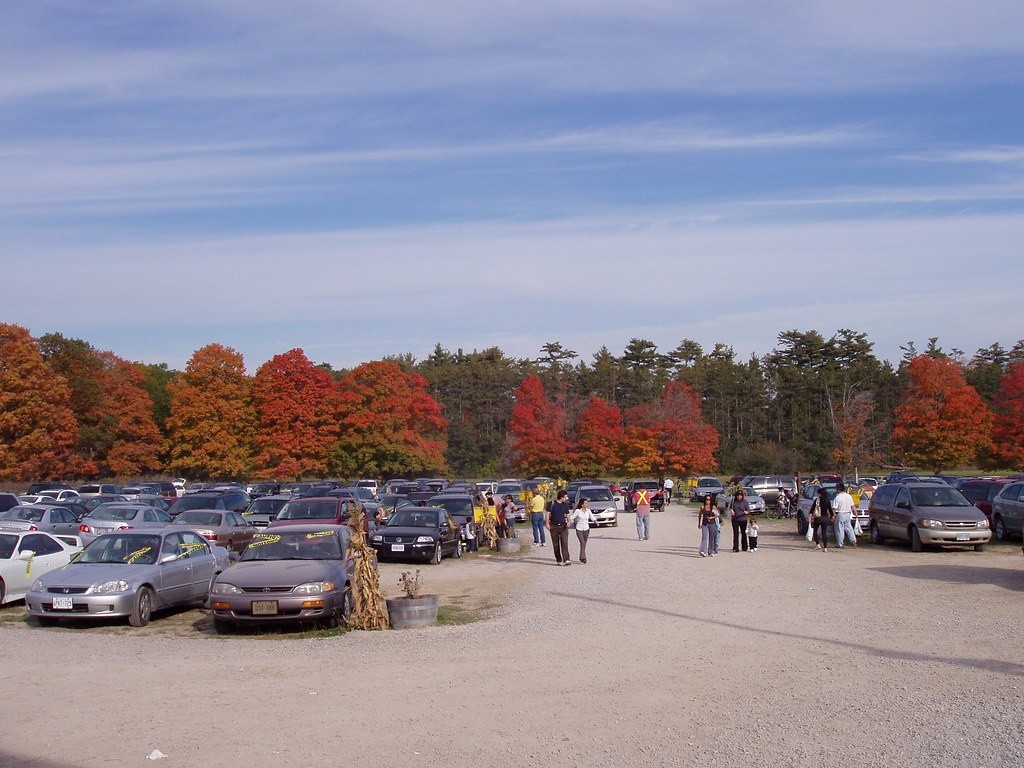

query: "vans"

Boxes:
[737, 474, 798, 502]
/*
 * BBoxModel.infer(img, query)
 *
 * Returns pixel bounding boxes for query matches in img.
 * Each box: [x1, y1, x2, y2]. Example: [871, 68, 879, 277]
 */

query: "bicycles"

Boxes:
[765, 495, 798, 521]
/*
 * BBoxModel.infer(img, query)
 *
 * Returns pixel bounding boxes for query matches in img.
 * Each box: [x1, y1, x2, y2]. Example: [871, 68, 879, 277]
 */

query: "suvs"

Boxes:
[622, 480, 664, 513]
[886, 472, 917, 484]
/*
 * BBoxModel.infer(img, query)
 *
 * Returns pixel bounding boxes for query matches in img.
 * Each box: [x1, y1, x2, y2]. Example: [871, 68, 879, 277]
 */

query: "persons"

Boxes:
[698, 493, 721, 557]
[373, 511, 387, 530]
[464, 516, 477, 552]
[484, 493, 517, 547]
[555, 476, 565, 490]
[527, 488, 546, 547]
[653, 477, 683, 505]
[418, 501, 428, 508]
[545, 491, 599, 565]
[732, 491, 759, 552]
[812, 473, 821, 483]
[809, 482, 858, 552]
[386, 483, 393, 495]
[776, 487, 795, 518]
[631, 487, 662, 541]
[730, 476, 738, 490]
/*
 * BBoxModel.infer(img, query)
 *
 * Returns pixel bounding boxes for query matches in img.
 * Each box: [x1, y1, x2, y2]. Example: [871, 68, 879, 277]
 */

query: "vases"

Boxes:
[385, 594, 439, 630]
[496, 538, 521, 554]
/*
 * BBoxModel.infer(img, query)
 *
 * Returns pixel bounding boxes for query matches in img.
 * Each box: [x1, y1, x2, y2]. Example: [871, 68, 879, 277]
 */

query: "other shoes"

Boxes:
[531, 543, 540, 548]
[852, 541, 857, 549]
[813, 545, 821, 550]
[835, 545, 844, 549]
[823, 548, 827, 552]
[540, 543, 546, 547]
[556, 562, 562, 565]
[563, 560, 571, 566]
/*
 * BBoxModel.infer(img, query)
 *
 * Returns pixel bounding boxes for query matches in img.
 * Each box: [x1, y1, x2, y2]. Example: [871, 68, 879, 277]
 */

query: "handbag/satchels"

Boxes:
[806, 519, 814, 541]
[853, 518, 863, 536]
[813, 498, 821, 517]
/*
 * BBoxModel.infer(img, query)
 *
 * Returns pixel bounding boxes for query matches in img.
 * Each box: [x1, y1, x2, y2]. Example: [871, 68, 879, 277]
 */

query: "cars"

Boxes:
[800, 473, 1024, 529]
[371, 506, 463, 565]
[210, 525, 378, 634]
[25, 528, 231, 629]
[689, 477, 724, 502]
[0, 491, 23, 512]
[796, 483, 872, 535]
[991, 481, 1024, 542]
[867, 481, 992, 553]
[715, 486, 766, 517]
[77, 504, 175, 549]
[0, 504, 81, 535]
[0, 529, 88, 608]
[163, 508, 261, 554]
[26, 477, 628, 538]
[424, 493, 485, 552]
[569, 485, 620, 528]
[266, 495, 370, 550]
[18, 495, 55, 505]
